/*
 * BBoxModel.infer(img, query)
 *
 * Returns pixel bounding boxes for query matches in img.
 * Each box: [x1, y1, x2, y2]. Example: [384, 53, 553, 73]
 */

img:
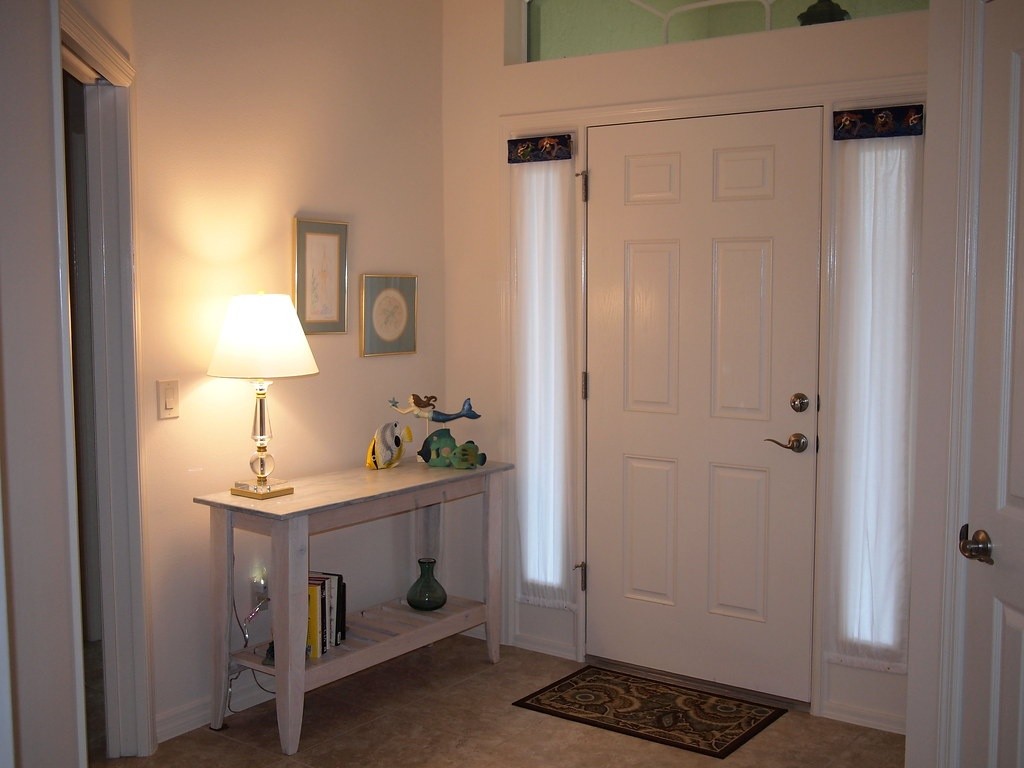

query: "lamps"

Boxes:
[207, 294, 319, 500]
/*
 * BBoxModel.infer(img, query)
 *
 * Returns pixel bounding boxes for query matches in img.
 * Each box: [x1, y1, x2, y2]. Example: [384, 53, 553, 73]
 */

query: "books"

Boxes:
[306, 569, 347, 660]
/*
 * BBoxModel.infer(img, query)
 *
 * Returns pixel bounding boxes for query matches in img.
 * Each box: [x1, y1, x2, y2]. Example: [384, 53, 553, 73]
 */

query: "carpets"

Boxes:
[511, 665, 788, 760]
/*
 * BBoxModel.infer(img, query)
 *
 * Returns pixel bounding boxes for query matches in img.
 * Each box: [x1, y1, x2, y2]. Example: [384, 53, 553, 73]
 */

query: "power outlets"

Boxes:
[251, 574, 269, 612]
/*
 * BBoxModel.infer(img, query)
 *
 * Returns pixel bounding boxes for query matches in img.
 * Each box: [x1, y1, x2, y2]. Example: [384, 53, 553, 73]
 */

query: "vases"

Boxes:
[407, 558, 448, 611]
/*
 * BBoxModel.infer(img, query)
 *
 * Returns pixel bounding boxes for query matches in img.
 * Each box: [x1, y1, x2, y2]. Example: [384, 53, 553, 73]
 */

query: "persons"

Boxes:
[385, 393, 482, 423]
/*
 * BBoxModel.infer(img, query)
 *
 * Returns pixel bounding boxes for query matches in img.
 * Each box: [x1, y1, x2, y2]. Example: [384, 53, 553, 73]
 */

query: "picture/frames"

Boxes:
[291, 216, 349, 335]
[359, 272, 417, 358]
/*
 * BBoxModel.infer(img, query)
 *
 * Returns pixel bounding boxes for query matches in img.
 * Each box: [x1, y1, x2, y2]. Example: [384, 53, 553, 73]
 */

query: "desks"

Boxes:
[194, 457, 515, 755]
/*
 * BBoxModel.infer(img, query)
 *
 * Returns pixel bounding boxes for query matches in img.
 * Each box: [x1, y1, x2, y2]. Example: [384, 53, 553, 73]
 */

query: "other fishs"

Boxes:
[417, 428, 488, 469]
[365, 420, 413, 471]
